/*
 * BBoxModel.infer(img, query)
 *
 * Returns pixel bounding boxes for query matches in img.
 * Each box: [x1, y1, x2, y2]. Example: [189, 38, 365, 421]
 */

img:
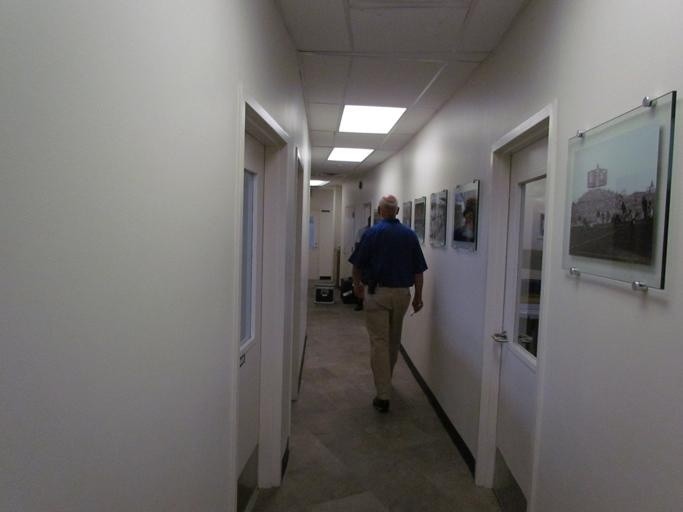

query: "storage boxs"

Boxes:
[313, 281, 336, 303]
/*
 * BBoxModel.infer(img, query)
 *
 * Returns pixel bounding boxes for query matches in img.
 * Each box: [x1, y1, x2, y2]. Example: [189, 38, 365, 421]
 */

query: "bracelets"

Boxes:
[351, 282, 360, 287]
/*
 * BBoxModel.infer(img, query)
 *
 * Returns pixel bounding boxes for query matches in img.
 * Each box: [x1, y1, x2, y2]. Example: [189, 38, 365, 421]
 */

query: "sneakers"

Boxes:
[372, 397, 390, 414]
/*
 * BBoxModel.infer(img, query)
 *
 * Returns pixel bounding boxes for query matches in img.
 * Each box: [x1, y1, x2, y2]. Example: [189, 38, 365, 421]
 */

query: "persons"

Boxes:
[352, 216, 371, 312]
[453, 198, 474, 243]
[347, 194, 429, 413]
